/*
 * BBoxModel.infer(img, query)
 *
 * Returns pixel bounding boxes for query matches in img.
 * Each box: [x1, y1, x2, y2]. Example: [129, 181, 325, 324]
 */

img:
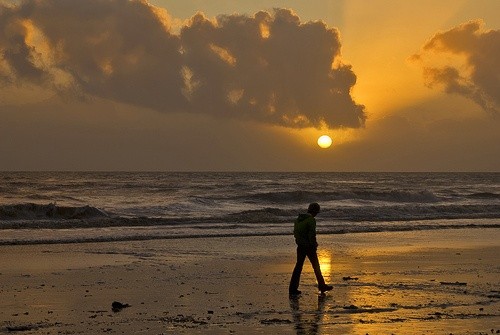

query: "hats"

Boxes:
[308, 202, 321, 213]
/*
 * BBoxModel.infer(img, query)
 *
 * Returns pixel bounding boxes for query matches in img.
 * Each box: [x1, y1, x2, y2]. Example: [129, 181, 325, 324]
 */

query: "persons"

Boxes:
[288, 203, 333, 296]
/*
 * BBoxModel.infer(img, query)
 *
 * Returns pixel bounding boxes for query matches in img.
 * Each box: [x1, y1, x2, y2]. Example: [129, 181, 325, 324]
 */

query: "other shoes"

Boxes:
[318, 284, 333, 290]
[289, 290, 301, 296]
